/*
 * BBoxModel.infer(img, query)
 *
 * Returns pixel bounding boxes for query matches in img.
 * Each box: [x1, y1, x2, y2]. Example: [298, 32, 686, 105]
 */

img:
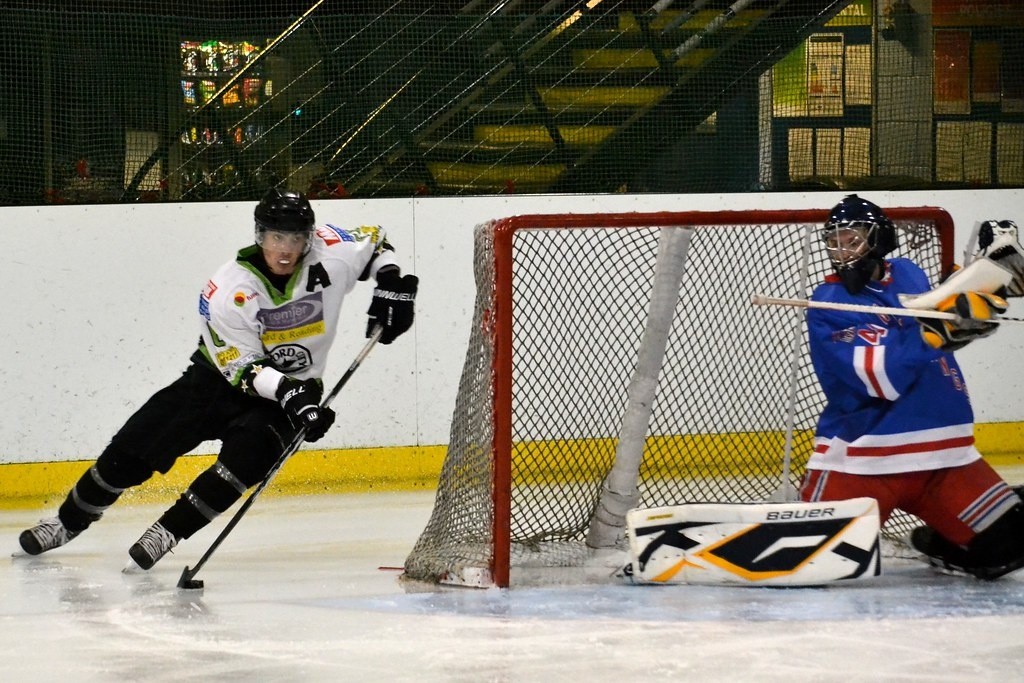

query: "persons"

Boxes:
[578, 193, 1024, 588]
[11, 186, 419, 572]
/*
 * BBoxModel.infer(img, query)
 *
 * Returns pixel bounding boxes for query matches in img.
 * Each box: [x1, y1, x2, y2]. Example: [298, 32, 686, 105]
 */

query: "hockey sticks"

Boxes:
[176, 323, 385, 591]
[750, 294, 1024, 326]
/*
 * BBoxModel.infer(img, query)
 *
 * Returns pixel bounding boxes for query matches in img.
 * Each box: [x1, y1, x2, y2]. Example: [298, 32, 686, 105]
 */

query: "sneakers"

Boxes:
[121, 521, 178, 572]
[10, 516, 77, 557]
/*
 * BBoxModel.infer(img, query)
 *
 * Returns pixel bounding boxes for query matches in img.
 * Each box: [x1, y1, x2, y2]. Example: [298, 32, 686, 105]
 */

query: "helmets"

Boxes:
[820, 194, 898, 296]
[254, 187, 315, 240]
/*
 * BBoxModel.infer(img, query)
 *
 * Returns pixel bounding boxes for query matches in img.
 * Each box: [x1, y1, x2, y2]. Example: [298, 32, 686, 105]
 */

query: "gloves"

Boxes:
[366, 272, 419, 344]
[922, 291, 1009, 350]
[276, 378, 335, 443]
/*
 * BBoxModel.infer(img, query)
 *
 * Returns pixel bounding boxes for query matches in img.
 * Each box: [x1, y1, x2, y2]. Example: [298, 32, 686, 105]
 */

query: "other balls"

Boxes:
[180, 578, 204, 590]
